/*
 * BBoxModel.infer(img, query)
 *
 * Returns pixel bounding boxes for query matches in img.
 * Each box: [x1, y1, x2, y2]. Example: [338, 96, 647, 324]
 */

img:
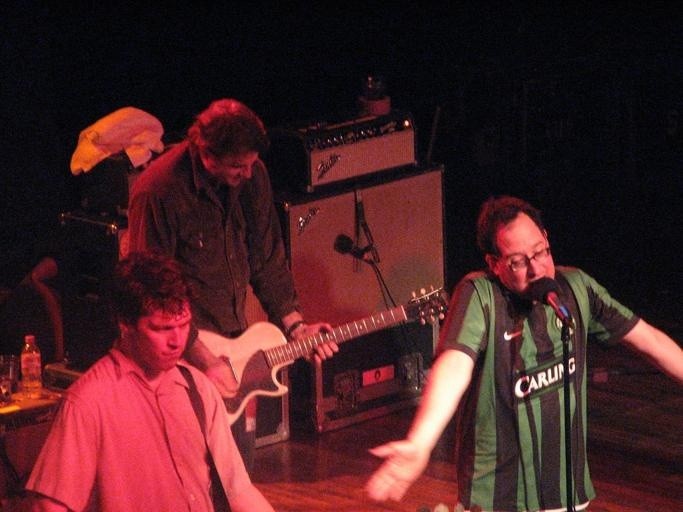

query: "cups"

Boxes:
[0, 354, 19, 401]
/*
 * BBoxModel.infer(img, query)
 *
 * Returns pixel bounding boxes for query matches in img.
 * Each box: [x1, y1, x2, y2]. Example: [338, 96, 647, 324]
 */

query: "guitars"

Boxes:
[195, 284, 449, 427]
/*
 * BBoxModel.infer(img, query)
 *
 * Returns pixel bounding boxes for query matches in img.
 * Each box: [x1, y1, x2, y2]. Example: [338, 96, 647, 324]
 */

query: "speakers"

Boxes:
[273, 160, 449, 329]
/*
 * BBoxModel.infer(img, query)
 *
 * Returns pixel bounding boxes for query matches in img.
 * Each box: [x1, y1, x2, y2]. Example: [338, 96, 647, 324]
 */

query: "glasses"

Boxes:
[493, 246, 550, 273]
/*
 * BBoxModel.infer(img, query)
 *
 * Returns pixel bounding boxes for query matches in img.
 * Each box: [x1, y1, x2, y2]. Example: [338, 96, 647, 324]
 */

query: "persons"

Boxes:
[356, 192, 681, 512]
[123, 96, 339, 474]
[17, 249, 277, 512]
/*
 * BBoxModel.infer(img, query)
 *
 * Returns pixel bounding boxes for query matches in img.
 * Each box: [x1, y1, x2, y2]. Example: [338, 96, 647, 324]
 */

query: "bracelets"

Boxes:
[284, 320, 306, 335]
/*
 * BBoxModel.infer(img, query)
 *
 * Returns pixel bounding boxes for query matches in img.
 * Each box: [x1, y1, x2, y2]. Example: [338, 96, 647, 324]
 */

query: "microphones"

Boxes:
[531, 277, 569, 322]
[335, 234, 370, 263]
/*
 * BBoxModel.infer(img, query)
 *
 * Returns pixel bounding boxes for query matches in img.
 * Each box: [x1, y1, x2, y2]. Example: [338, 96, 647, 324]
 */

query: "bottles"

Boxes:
[20, 336, 44, 402]
